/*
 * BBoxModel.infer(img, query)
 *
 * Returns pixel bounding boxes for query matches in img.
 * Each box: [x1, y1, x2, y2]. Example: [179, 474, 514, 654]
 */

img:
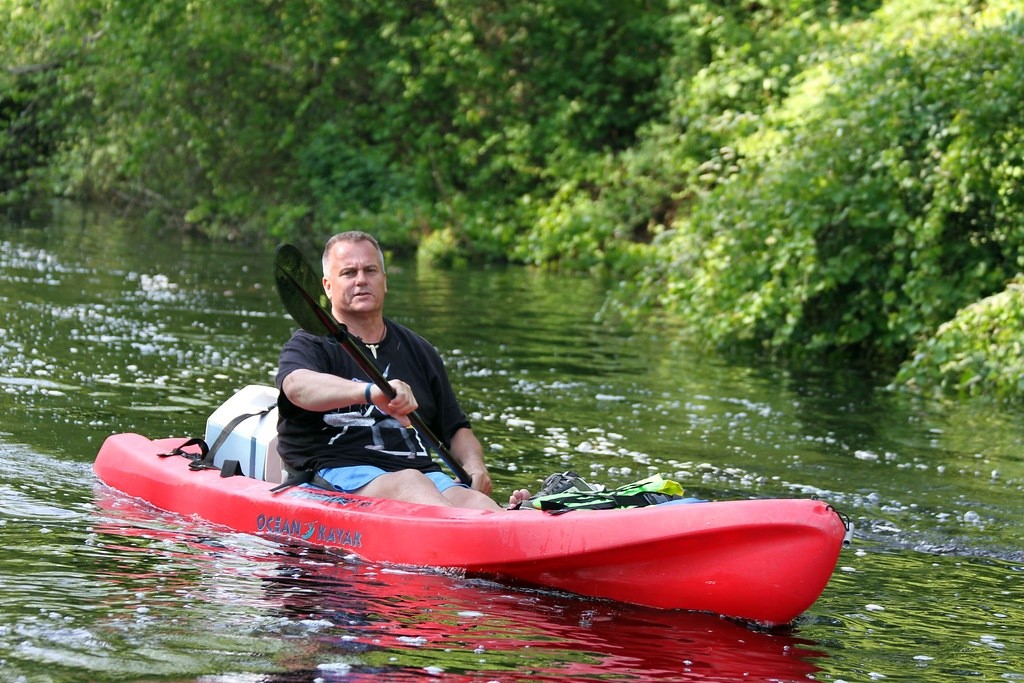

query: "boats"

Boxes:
[94, 426, 847, 629]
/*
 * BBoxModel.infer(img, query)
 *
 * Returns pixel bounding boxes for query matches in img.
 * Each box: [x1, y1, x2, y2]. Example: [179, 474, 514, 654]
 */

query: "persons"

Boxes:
[275, 230, 530, 511]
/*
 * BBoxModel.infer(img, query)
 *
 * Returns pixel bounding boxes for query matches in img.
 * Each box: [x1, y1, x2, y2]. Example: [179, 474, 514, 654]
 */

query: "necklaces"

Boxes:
[365, 324, 385, 359]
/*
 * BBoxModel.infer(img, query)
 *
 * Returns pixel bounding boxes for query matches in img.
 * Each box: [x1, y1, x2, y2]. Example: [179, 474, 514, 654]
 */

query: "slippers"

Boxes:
[542, 493, 617, 515]
[536, 481, 607, 510]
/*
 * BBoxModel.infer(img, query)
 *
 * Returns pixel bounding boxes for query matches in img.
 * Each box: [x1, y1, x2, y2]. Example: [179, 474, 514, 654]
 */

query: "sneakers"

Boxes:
[522, 470, 578, 507]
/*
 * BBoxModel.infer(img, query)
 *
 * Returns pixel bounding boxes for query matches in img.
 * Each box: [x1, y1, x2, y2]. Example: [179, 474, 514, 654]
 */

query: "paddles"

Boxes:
[272, 242, 507, 511]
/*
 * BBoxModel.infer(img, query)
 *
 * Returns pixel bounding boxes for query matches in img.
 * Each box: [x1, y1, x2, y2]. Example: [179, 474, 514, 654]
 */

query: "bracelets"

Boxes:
[365, 383, 375, 405]
